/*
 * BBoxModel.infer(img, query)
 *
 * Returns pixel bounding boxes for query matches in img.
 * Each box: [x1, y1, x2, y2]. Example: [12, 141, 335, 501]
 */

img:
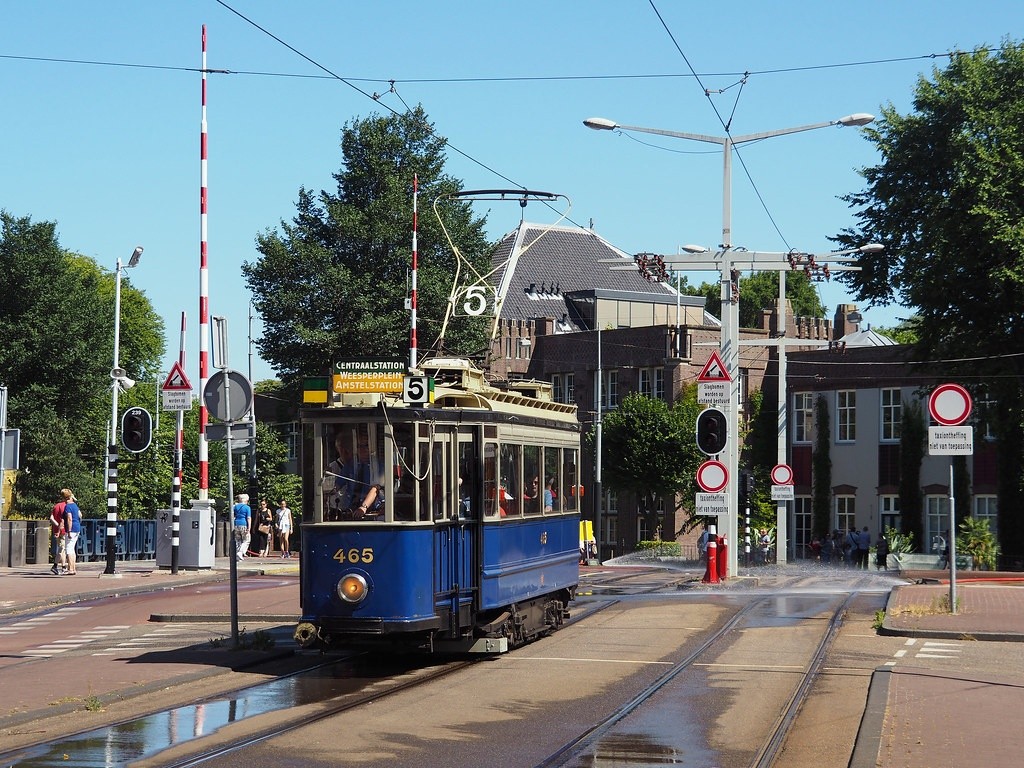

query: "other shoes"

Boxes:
[236, 552, 244, 561]
[264, 553, 267, 557]
[259, 554, 263, 558]
[51, 567, 60, 575]
[62, 570, 76, 575]
[286, 553, 290, 557]
[62, 568, 67, 573]
[281, 554, 285, 559]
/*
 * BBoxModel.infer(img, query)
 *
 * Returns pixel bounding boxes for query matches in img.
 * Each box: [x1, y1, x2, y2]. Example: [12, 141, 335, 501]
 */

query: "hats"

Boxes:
[71, 494, 78, 501]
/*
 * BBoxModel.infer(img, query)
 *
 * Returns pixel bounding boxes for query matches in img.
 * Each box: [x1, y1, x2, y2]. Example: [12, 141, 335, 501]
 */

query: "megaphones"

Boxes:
[110, 367, 126, 379]
[120, 376, 135, 389]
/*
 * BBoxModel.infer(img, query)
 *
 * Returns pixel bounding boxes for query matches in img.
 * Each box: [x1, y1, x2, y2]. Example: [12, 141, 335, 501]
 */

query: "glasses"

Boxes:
[261, 504, 266, 506]
[528, 481, 539, 485]
[357, 444, 368, 450]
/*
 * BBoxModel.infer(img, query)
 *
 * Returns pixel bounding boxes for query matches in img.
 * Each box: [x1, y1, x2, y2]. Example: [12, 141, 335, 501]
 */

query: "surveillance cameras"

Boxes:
[129, 246, 144, 266]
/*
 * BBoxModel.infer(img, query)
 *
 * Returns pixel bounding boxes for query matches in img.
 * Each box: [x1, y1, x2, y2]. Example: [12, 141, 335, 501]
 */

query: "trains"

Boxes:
[294, 189, 586, 656]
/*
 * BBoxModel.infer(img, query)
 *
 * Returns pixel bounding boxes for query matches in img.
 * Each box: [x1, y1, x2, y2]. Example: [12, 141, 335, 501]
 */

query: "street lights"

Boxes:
[682, 244, 886, 566]
[583, 113, 877, 575]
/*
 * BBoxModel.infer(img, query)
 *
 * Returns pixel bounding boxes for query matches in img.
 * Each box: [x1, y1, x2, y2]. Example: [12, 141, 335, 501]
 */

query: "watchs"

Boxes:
[361, 504, 368, 509]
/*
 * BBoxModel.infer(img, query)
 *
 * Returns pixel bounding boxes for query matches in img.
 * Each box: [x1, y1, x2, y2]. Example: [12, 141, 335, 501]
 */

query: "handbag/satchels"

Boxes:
[275, 521, 280, 534]
[258, 524, 269, 534]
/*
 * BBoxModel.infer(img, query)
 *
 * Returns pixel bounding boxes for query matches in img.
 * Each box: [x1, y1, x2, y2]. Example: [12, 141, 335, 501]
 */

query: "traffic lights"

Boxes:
[121, 406, 152, 453]
[695, 409, 727, 455]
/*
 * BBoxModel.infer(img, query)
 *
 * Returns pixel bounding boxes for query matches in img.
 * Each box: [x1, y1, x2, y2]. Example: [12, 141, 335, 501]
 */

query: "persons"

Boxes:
[49, 488, 83, 575]
[700, 523, 710, 581]
[875, 533, 890, 571]
[526, 472, 582, 510]
[253, 499, 272, 557]
[234, 494, 252, 562]
[943, 530, 950, 569]
[757, 528, 771, 565]
[808, 525, 872, 571]
[322, 428, 386, 522]
[274, 500, 293, 559]
